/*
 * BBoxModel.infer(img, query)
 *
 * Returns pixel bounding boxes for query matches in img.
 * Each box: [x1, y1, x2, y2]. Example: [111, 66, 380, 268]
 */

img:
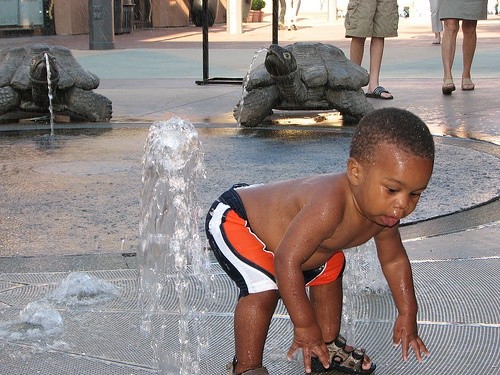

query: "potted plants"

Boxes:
[247, 0, 266, 22]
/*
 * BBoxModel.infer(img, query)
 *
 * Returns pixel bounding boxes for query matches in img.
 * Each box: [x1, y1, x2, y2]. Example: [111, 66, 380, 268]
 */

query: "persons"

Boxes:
[345, 0, 399, 100]
[430, 1, 449, 44]
[438, 0, 490, 94]
[205, 108, 435, 375]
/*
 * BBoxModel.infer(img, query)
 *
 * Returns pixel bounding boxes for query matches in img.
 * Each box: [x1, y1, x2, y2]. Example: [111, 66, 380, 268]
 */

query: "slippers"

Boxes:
[365, 87, 393, 100]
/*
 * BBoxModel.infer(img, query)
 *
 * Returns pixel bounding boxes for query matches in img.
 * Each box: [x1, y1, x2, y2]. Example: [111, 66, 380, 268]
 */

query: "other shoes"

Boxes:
[432, 37, 441, 44]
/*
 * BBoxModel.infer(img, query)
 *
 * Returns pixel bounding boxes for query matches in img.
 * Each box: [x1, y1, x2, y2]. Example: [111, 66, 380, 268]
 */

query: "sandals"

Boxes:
[226, 355, 269, 375]
[442, 80, 455, 95]
[462, 80, 475, 91]
[311, 335, 376, 375]
